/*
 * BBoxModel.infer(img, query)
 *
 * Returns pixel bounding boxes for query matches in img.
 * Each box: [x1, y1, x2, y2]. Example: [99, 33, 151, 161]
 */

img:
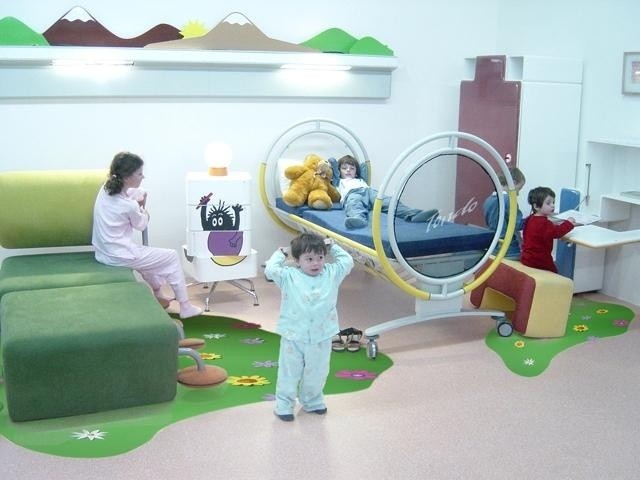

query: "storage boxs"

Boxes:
[188, 205, 254, 232]
[187, 232, 252, 256]
[187, 171, 252, 205]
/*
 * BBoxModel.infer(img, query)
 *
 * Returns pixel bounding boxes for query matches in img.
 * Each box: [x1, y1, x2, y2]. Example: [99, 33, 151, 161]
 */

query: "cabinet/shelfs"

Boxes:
[181, 245, 263, 312]
[453, 54, 584, 221]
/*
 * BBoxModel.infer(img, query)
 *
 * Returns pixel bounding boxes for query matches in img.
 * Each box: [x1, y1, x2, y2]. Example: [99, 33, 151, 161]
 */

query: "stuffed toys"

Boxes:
[280, 151, 341, 211]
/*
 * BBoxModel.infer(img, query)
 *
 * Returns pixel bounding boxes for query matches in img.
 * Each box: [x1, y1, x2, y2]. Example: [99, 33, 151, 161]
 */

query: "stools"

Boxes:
[471, 248, 573, 337]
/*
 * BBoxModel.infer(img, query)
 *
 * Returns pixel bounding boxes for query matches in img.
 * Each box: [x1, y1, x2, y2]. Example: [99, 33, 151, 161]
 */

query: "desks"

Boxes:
[555, 189, 640, 298]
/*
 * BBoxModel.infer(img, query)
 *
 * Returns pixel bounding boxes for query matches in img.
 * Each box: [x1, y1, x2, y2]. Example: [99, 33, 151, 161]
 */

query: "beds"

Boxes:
[0, 172, 180, 422]
[273, 151, 516, 357]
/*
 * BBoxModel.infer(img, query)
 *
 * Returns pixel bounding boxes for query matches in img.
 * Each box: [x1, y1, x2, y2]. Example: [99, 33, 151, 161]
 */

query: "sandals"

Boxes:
[333, 327, 363, 352]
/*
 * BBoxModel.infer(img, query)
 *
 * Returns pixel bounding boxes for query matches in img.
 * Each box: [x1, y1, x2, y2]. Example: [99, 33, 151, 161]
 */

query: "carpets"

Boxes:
[0, 311, 395, 459]
[483, 295, 636, 381]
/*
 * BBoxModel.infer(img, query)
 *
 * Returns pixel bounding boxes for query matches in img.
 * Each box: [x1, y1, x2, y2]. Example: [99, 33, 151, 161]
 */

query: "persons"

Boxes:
[89, 150, 204, 320]
[328, 154, 442, 230]
[263, 232, 355, 424]
[518, 187, 577, 274]
[199, 195, 243, 231]
[483, 167, 527, 264]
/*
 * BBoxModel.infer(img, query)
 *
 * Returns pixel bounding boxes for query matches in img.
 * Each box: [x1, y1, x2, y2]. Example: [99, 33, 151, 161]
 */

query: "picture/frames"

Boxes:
[621, 51, 640, 97]
